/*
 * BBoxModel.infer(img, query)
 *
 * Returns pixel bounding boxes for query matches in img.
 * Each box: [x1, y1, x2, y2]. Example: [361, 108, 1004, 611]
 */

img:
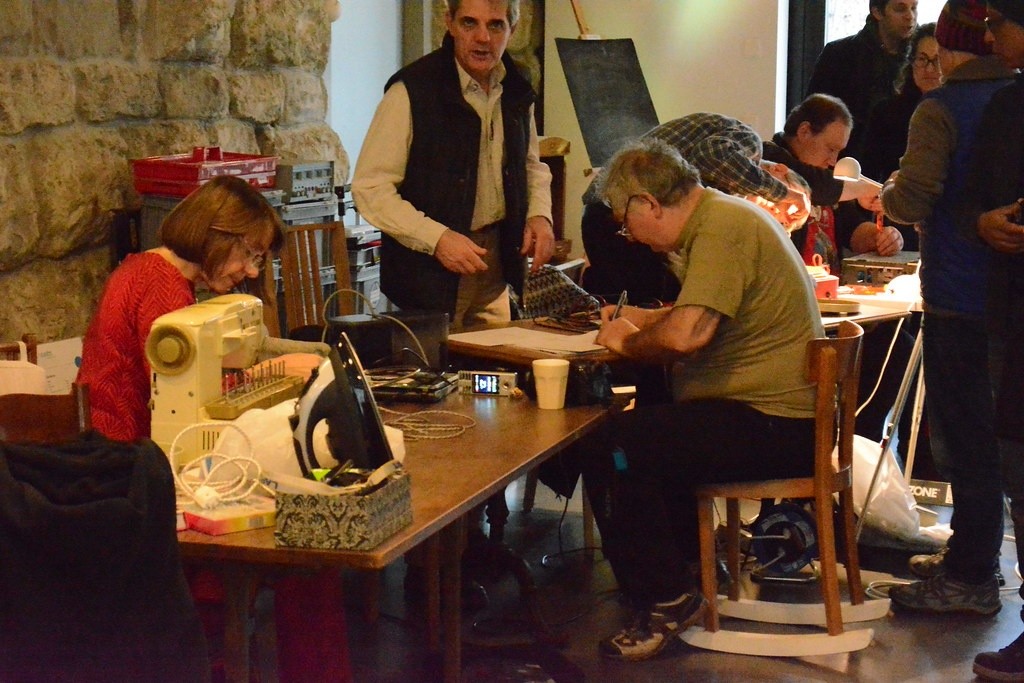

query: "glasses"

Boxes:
[615, 194, 639, 237]
[907, 55, 941, 69]
[984, 16, 1008, 35]
[237, 234, 266, 272]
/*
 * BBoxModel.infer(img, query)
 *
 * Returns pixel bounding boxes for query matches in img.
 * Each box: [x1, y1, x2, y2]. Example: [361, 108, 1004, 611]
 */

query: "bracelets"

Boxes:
[881, 179, 895, 194]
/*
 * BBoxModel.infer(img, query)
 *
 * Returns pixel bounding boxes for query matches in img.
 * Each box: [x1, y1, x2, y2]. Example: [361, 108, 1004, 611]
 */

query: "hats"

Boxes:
[934, 0, 993, 57]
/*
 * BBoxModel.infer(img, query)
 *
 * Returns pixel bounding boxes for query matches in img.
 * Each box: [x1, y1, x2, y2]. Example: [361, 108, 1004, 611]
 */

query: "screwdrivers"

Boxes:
[876, 211, 883, 236]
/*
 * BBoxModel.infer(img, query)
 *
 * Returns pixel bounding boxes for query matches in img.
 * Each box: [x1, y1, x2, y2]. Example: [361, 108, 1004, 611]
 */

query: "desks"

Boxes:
[447, 306, 634, 561]
[186, 393, 633, 683]
[822, 277, 914, 329]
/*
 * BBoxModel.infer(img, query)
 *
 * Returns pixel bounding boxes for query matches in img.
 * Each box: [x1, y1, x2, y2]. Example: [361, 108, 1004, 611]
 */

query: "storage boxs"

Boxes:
[128, 138, 403, 344]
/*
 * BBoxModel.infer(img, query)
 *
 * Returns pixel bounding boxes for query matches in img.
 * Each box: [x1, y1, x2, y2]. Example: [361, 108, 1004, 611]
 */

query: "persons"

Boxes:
[75, 174, 359, 682]
[807, 1, 923, 253]
[853, 0, 1024, 618]
[758, 94, 906, 282]
[869, 20, 948, 188]
[941, 1, 1024, 683]
[581, 111, 815, 416]
[353, 2, 559, 623]
[592, 139, 839, 658]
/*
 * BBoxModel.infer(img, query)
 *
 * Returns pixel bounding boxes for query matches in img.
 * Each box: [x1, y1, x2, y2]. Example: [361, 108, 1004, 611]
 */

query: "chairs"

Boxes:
[0, 378, 95, 447]
[684, 315, 866, 642]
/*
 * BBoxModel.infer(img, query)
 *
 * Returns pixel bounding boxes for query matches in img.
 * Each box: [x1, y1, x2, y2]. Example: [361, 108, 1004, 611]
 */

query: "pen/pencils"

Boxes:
[610, 289, 627, 320]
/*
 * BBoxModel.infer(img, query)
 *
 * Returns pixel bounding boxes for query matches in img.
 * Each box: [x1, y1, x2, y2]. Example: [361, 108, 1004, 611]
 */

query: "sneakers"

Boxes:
[597, 588, 710, 663]
[888, 568, 1003, 620]
[972, 640, 1024, 682]
[907, 547, 1006, 586]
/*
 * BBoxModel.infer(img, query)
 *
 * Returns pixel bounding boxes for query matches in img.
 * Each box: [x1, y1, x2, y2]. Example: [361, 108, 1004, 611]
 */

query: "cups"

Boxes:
[533, 356, 569, 411]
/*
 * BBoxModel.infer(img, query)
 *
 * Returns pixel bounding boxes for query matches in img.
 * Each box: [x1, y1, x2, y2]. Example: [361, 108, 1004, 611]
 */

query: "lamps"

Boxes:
[831, 155, 886, 191]
